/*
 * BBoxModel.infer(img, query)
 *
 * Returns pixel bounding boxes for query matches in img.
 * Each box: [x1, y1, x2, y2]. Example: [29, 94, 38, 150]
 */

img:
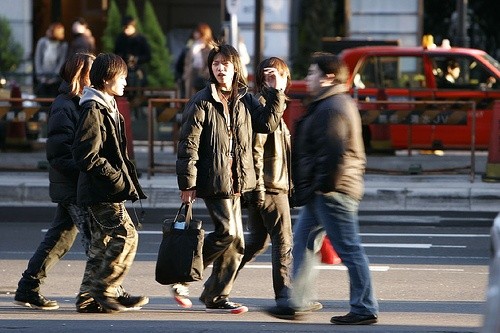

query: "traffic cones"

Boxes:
[482, 100, 500, 183]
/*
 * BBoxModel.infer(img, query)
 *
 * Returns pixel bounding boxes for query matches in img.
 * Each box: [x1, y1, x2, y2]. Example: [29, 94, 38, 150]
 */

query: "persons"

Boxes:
[437, 60, 496, 90]
[74, 51, 149, 313]
[167, 44, 285, 314]
[199, 57, 323, 312]
[33, 15, 250, 139]
[264, 52, 379, 325]
[13, 52, 143, 311]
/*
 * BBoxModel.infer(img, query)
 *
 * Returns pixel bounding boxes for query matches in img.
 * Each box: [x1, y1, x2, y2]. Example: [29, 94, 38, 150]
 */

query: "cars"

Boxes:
[282, 35, 500, 155]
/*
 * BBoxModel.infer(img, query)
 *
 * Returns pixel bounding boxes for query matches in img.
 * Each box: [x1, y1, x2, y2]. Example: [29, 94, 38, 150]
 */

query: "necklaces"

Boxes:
[220, 91, 232, 105]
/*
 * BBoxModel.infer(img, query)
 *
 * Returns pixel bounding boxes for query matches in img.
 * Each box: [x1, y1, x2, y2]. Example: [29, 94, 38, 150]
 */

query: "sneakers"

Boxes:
[116, 291, 148, 308]
[14, 289, 59, 310]
[170, 283, 192, 309]
[206, 300, 248, 313]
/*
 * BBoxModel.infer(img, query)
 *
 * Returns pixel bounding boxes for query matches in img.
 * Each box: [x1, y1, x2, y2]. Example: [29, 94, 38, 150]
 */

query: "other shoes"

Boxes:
[330, 312, 378, 325]
[304, 301, 322, 310]
[77, 296, 126, 313]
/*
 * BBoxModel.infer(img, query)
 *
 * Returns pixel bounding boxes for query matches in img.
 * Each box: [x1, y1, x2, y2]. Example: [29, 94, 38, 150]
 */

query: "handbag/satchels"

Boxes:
[155, 201, 204, 285]
